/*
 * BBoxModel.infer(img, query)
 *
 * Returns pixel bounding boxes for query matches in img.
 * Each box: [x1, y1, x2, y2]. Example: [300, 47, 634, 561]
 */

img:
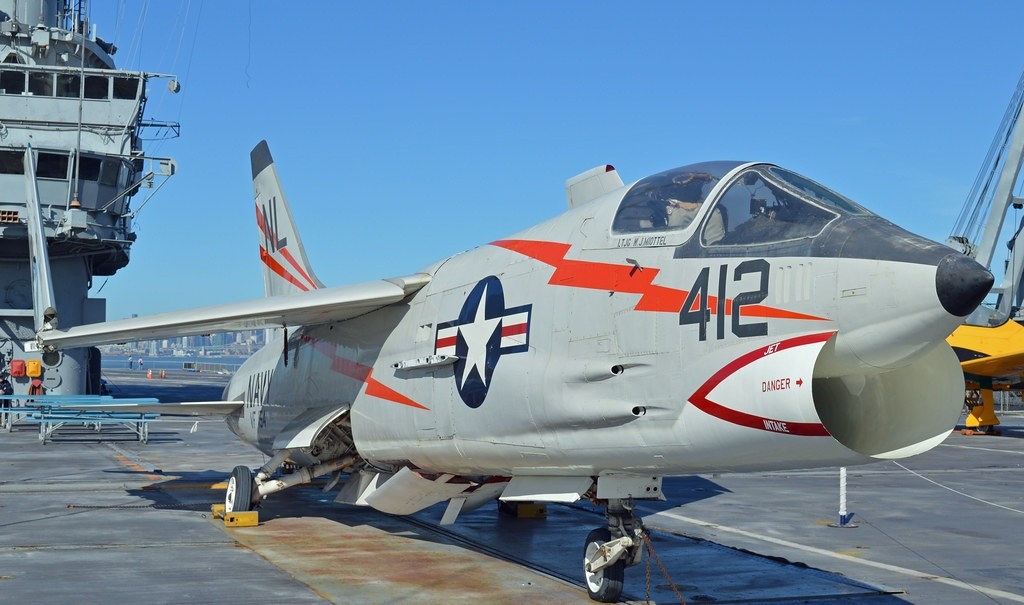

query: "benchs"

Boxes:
[0, 395, 161, 445]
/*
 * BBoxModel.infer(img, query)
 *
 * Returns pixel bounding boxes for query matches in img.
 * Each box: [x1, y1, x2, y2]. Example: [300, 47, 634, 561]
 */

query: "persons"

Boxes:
[138, 357, 143, 370]
[128, 355, 132, 369]
[635, 172, 729, 245]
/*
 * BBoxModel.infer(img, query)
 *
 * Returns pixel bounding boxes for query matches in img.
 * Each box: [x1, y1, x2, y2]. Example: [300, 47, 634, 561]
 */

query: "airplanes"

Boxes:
[23, 137, 995, 603]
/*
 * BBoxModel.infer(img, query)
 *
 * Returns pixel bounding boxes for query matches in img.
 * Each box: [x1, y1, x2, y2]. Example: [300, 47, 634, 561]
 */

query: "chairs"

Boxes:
[667, 202, 725, 246]
